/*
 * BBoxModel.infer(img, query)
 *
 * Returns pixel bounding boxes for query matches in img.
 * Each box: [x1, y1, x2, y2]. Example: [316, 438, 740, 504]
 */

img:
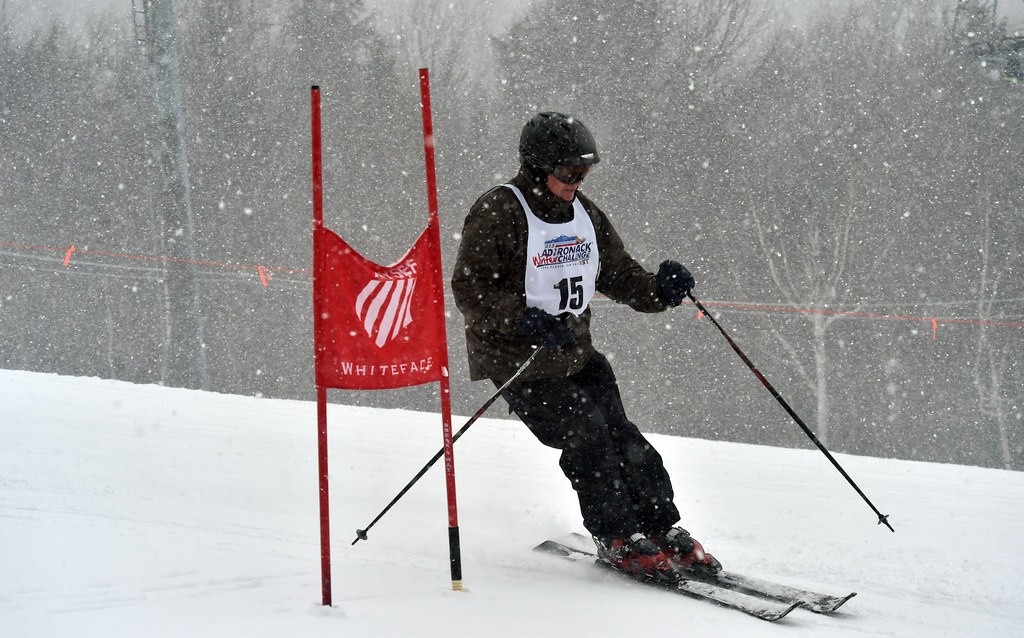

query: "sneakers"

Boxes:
[595, 528, 680, 585]
[656, 528, 720, 576]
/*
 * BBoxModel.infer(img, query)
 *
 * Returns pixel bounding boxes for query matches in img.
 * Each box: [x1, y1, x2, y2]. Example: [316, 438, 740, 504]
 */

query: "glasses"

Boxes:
[551, 163, 589, 183]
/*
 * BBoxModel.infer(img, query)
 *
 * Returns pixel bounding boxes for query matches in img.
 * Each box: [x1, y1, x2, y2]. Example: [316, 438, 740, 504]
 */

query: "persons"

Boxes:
[451, 111, 724, 583]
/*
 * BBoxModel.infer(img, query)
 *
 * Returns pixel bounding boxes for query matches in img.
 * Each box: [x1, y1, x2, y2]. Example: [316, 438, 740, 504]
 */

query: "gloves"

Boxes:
[656, 258, 694, 307]
[526, 309, 575, 353]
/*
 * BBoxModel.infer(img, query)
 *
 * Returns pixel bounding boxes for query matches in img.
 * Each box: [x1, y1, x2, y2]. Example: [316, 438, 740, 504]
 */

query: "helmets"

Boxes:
[519, 114, 600, 183]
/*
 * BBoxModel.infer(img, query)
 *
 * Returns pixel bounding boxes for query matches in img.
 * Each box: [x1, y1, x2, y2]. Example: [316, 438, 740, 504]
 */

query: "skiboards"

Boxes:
[532, 534, 861, 623]
